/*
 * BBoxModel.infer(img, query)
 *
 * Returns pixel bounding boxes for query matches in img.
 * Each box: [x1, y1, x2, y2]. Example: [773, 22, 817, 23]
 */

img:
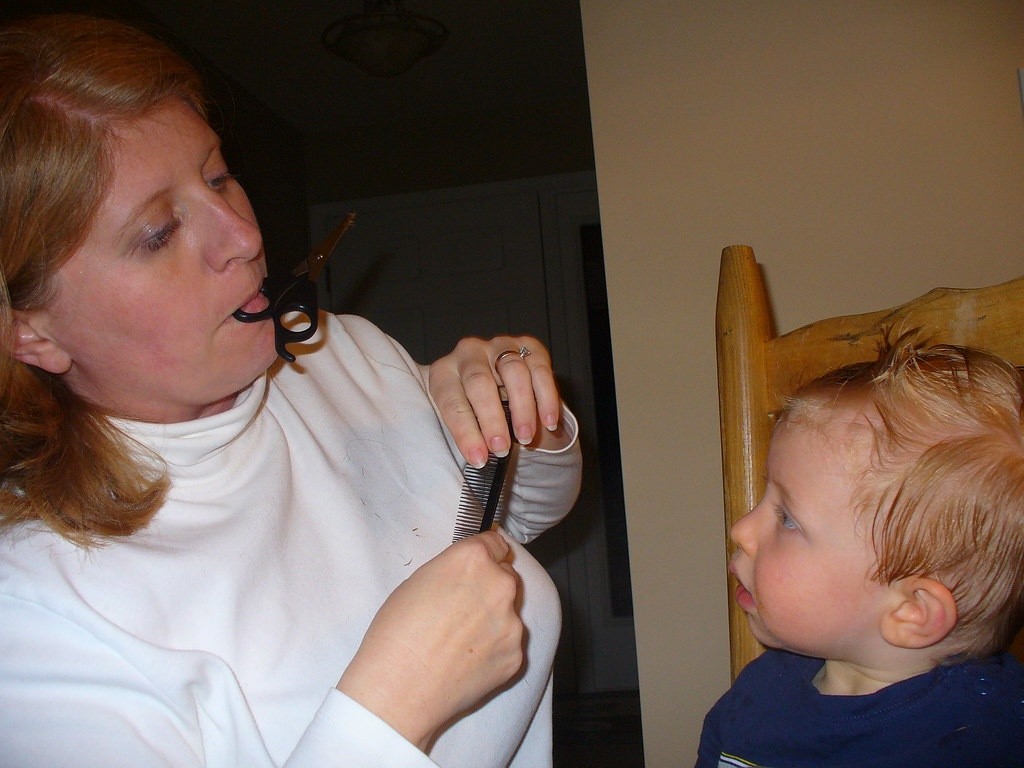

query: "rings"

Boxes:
[495, 346, 530, 372]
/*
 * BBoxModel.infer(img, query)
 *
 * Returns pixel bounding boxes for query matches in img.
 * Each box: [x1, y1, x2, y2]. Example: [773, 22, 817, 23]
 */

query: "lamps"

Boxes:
[322, 1, 451, 78]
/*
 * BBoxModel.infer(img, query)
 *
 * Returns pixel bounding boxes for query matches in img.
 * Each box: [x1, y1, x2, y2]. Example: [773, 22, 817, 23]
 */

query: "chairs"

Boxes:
[716, 246, 1023, 689]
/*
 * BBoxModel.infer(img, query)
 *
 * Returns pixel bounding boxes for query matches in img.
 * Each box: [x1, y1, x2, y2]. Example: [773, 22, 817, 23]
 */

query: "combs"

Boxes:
[453, 405, 515, 543]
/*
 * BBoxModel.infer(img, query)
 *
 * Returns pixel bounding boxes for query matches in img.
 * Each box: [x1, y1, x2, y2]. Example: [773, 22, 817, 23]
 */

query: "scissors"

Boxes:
[232, 209, 358, 363]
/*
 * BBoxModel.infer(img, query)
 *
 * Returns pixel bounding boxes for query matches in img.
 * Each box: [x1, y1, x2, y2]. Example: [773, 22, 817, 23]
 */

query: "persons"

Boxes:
[0, 12, 581, 768]
[692, 320, 1024, 768]
